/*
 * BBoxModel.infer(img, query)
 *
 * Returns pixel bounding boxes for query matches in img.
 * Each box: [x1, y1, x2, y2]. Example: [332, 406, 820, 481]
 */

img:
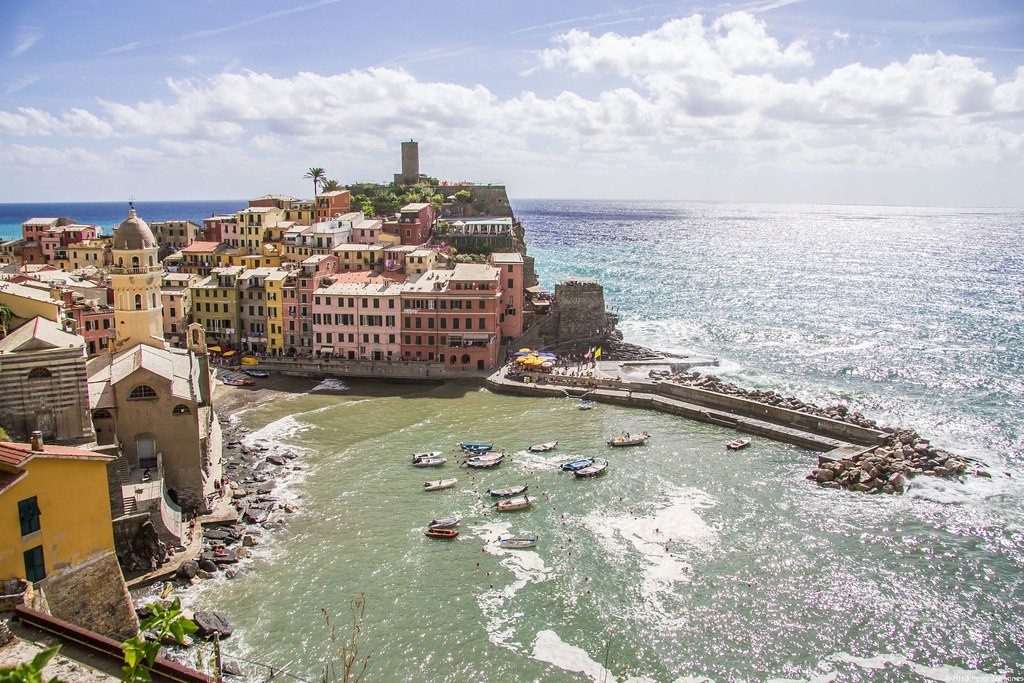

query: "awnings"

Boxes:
[514, 348, 557, 372]
[223, 350, 236, 357]
[207, 346, 221, 351]
[526, 286, 548, 293]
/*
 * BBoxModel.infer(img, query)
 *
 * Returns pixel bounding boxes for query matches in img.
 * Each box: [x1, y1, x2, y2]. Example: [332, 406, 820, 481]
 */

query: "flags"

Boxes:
[584, 347, 600, 358]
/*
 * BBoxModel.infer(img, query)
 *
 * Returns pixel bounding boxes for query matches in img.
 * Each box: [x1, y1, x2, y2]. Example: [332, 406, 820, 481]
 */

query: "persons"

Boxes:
[129, 471, 231, 574]
[523, 354, 597, 386]
[210, 351, 339, 367]
[417, 456, 422, 463]
[617, 374, 621, 380]
[596, 328, 600, 337]
[432, 520, 437, 525]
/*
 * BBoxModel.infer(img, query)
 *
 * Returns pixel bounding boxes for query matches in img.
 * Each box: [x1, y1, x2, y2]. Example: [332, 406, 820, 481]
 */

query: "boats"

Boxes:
[726, 436, 752, 450]
[492, 494, 537, 512]
[579, 398, 593, 410]
[606, 430, 652, 446]
[493, 535, 538, 548]
[459, 442, 505, 468]
[483, 483, 528, 498]
[427, 514, 463, 529]
[527, 438, 559, 453]
[411, 450, 447, 467]
[244, 369, 270, 377]
[423, 476, 458, 491]
[557, 455, 594, 471]
[575, 460, 608, 478]
[221, 374, 257, 386]
[423, 528, 459, 539]
